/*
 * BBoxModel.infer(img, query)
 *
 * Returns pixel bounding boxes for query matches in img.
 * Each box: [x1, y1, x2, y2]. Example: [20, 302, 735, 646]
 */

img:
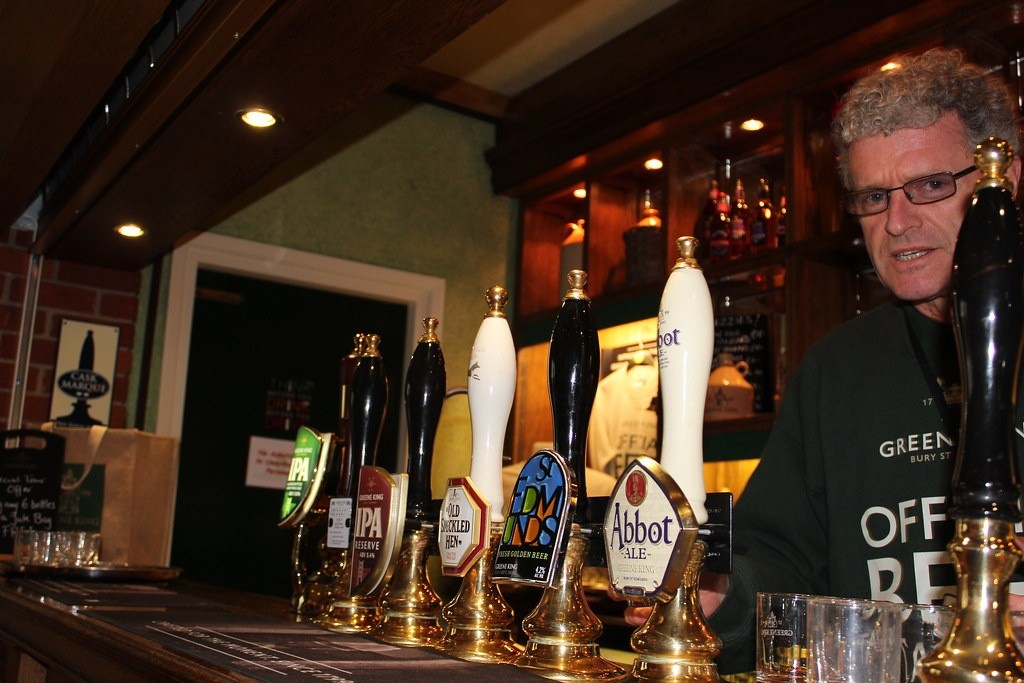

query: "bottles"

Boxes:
[557, 175, 786, 305]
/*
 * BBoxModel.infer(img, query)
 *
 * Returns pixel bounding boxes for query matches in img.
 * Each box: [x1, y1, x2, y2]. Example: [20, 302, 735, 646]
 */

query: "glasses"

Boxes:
[844, 165, 981, 218]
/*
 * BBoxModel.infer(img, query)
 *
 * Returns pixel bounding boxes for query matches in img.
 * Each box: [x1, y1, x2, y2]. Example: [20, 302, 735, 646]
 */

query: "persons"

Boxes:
[607, 44, 1022, 654]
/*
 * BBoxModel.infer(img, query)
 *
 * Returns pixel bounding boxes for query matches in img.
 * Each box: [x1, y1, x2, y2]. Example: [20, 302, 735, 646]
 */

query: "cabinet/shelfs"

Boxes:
[484, 0, 1023, 463]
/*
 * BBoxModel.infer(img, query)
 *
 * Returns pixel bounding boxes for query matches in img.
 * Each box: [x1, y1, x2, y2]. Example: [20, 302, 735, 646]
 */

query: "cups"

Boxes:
[755, 592, 810, 682]
[13, 530, 101, 568]
[900, 603, 957, 683]
[805, 596, 899, 683]
